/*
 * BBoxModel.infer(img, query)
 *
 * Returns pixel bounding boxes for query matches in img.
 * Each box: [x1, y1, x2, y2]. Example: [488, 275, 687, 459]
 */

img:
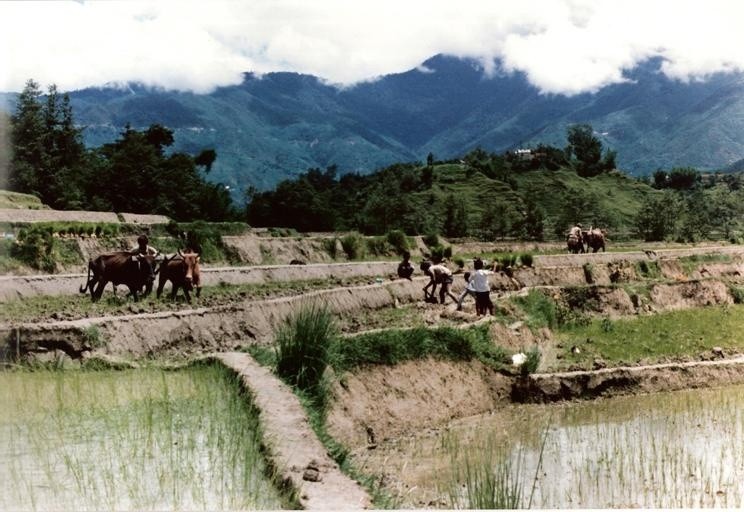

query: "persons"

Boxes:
[130, 234, 158, 257]
[420, 261, 459, 304]
[567, 222, 585, 253]
[398, 251, 414, 281]
[457, 257, 498, 316]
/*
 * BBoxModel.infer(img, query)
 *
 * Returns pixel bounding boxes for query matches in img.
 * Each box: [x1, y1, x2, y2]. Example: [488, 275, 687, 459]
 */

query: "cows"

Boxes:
[156, 248, 202, 305]
[583, 230, 606, 253]
[79, 251, 161, 302]
